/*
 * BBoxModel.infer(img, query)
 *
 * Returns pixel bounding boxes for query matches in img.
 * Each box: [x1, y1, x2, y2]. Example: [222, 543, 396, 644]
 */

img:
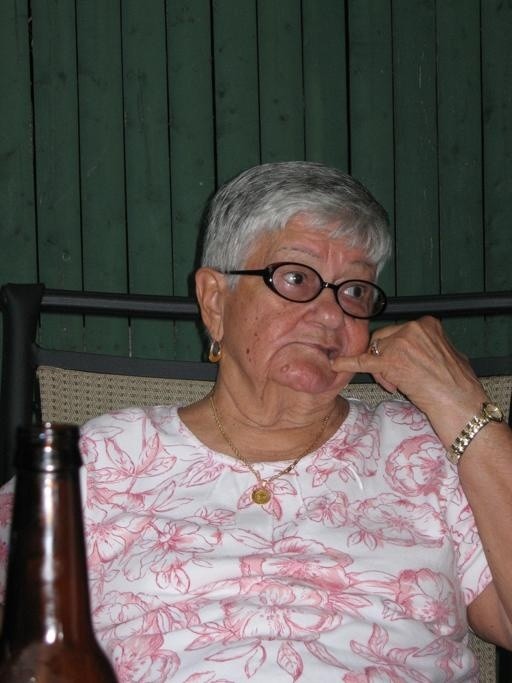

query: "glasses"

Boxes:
[225, 261, 387, 319]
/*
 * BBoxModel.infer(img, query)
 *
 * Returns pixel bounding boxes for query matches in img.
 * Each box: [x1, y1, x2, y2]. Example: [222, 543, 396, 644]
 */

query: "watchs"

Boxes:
[446, 394, 504, 461]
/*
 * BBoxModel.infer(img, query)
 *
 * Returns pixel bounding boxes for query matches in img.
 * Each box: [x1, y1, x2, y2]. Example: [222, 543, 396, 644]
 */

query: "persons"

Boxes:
[0, 160, 512, 682]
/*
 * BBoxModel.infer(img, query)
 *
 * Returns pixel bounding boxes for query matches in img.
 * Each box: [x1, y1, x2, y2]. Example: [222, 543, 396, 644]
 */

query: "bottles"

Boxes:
[1, 420, 119, 683]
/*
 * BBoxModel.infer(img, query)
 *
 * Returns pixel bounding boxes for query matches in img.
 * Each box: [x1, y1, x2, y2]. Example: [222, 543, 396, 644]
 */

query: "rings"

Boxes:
[369, 336, 383, 357]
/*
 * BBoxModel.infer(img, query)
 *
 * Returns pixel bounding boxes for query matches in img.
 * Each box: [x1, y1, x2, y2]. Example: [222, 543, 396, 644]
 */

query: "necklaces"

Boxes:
[204, 392, 335, 504]
